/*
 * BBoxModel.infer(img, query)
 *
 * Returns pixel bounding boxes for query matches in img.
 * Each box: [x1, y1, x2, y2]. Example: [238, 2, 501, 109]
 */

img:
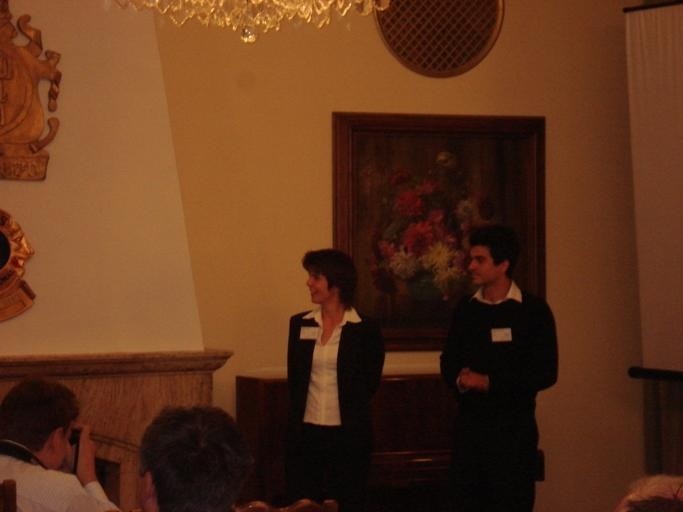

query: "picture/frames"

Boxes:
[323, 103, 550, 357]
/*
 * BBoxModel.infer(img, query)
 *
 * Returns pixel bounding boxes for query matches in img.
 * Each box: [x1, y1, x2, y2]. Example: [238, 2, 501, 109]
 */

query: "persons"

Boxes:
[287, 250, 383, 506]
[137, 406, 254, 511]
[440, 233, 559, 512]
[0, 378, 117, 511]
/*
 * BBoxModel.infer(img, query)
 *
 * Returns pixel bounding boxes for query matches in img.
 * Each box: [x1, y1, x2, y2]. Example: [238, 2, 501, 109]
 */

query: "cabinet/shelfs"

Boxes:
[228, 368, 550, 511]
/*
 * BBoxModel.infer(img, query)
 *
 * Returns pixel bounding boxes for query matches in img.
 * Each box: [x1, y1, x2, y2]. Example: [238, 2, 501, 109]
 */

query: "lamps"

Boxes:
[127, 1, 393, 46]
